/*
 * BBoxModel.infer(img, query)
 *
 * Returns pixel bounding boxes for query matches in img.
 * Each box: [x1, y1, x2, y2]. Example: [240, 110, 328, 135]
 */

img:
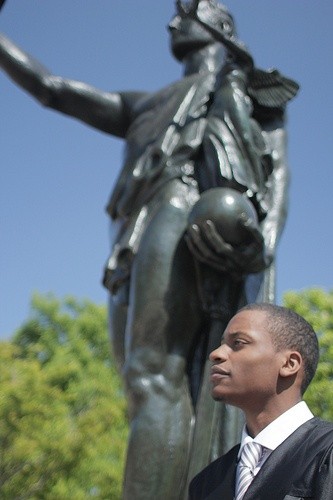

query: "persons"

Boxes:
[188, 304, 333, 500]
[0, 0, 299, 500]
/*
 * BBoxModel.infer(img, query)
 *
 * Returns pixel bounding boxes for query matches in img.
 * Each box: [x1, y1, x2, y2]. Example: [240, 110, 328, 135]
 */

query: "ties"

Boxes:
[235, 442, 263, 500]
